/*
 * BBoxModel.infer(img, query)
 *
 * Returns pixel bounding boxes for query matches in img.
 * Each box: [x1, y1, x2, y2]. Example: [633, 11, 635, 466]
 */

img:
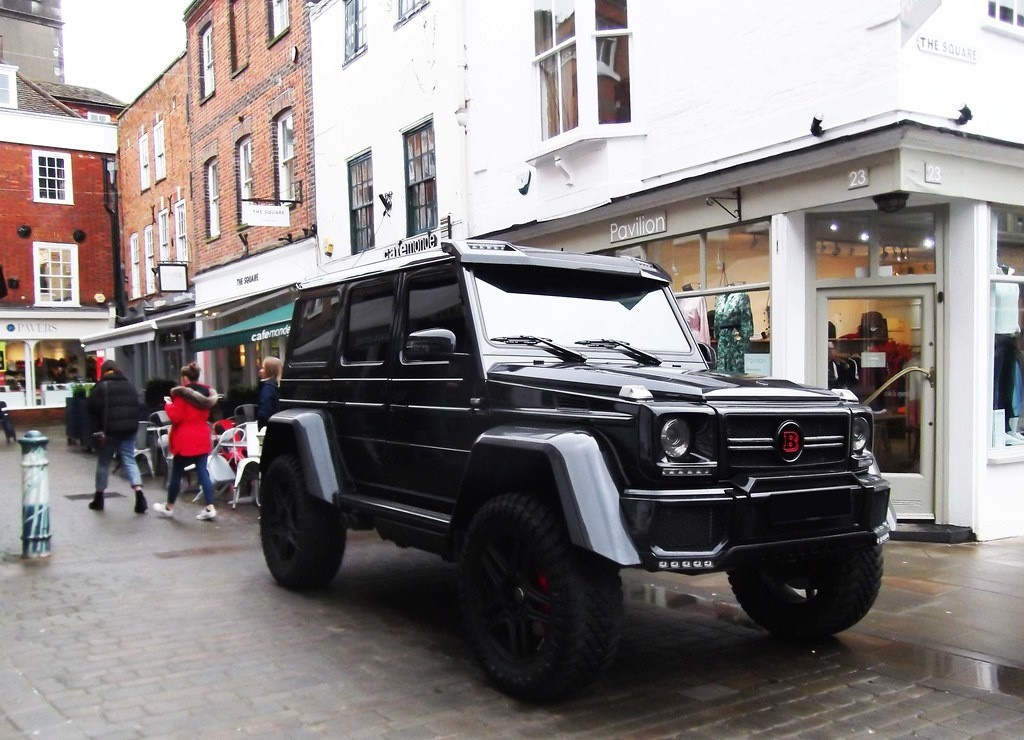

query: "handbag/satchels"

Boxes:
[92, 432, 106, 449]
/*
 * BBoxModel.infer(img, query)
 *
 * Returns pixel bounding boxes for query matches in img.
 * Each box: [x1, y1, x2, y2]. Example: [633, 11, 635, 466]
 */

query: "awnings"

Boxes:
[80, 277, 308, 351]
[187, 298, 304, 357]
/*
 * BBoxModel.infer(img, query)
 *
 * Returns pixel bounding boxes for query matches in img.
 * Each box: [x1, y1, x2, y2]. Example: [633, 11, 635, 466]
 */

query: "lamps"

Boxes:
[873, 193, 909, 213]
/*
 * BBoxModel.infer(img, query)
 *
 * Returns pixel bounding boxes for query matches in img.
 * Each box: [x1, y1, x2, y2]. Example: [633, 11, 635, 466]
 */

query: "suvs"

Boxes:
[259, 237, 897, 707]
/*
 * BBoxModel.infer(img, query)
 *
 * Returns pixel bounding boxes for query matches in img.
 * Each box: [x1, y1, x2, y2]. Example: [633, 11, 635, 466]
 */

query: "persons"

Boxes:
[152, 363, 217, 520]
[250, 355, 283, 433]
[989, 260, 1024, 447]
[83, 359, 148, 515]
[677, 282, 753, 374]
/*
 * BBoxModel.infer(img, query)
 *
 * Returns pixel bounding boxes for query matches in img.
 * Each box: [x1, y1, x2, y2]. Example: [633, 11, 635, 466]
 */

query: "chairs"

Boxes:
[91, 404, 260, 510]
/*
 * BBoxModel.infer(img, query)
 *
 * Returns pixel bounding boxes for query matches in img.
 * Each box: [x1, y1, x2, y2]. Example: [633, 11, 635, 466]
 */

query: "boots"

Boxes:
[89, 492, 104, 509]
[135, 491, 147, 512]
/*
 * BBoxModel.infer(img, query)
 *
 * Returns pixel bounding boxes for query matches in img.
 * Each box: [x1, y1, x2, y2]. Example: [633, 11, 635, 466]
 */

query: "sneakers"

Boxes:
[196, 506, 216, 519]
[153, 502, 172, 517]
